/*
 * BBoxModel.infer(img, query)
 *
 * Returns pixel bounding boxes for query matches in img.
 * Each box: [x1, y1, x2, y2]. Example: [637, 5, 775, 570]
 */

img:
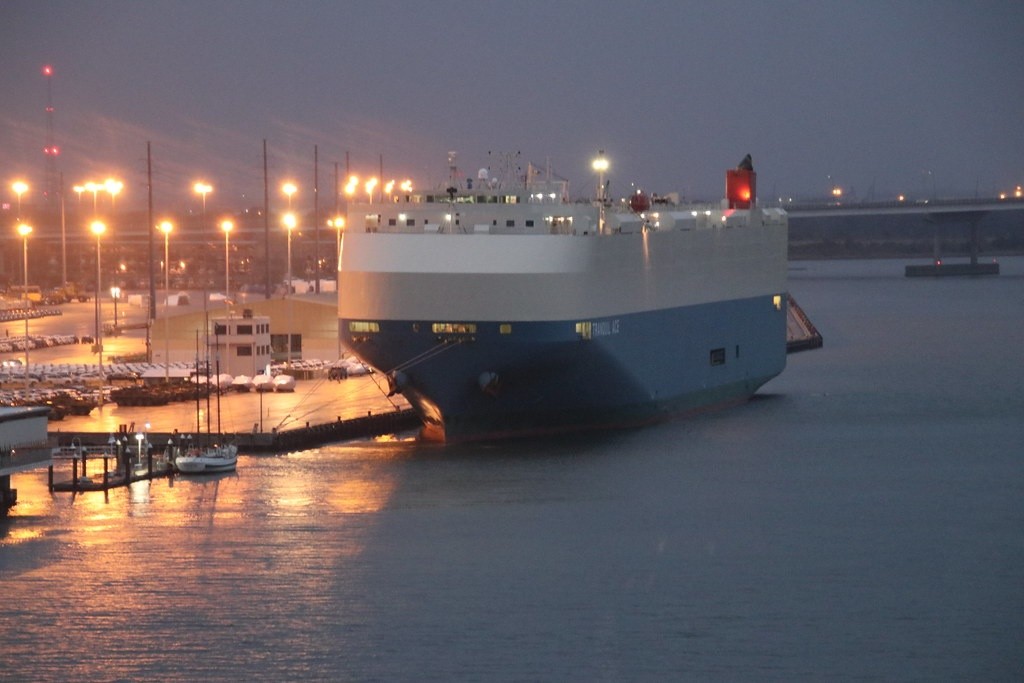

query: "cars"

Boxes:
[0, 334, 94, 352]
[1, 386, 123, 419]
[1, 371, 138, 387]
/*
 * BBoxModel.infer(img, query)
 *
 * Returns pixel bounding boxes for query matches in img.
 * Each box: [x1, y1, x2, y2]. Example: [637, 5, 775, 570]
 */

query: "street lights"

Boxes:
[283, 213, 295, 374]
[94, 223, 105, 419]
[343, 176, 413, 204]
[144, 421, 150, 458]
[284, 183, 296, 212]
[69, 437, 81, 478]
[20, 224, 30, 404]
[135, 426, 143, 464]
[194, 182, 212, 378]
[591, 157, 609, 236]
[222, 220, 233, 376]
[111, 286, 119, 337]
[108, 436, 115, 474]
[162, 223, 173, 384]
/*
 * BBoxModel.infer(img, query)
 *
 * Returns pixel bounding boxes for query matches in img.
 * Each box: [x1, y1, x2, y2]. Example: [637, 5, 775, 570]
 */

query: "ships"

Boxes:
[336, 153, 789, 438]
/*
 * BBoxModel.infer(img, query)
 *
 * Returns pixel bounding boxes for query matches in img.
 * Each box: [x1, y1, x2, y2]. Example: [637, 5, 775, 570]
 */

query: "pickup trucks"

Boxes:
[8, 282, 91, 305]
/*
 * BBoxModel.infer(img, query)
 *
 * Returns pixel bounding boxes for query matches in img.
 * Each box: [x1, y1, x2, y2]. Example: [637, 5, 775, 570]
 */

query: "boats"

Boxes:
[175, 444, 237, 472]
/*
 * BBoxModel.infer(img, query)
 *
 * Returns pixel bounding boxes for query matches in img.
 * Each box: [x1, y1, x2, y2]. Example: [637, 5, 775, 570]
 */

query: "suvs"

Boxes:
[109, 383, 212, 406]
